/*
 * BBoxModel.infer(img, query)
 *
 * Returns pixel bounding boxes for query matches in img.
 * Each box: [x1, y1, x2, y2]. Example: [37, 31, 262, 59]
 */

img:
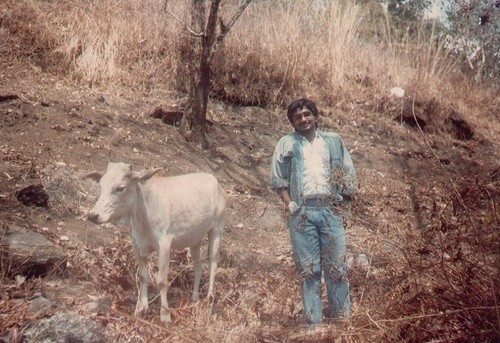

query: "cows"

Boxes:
[79, 161, 226, 325]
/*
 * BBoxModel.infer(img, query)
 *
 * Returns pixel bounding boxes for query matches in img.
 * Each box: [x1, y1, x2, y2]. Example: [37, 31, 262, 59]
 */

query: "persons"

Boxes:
[270, 98, 357, 332]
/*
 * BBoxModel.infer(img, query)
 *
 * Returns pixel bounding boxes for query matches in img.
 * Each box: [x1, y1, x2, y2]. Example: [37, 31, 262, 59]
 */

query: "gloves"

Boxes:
[335, 193, 344, 203]
[286, 200, 298, 216]
